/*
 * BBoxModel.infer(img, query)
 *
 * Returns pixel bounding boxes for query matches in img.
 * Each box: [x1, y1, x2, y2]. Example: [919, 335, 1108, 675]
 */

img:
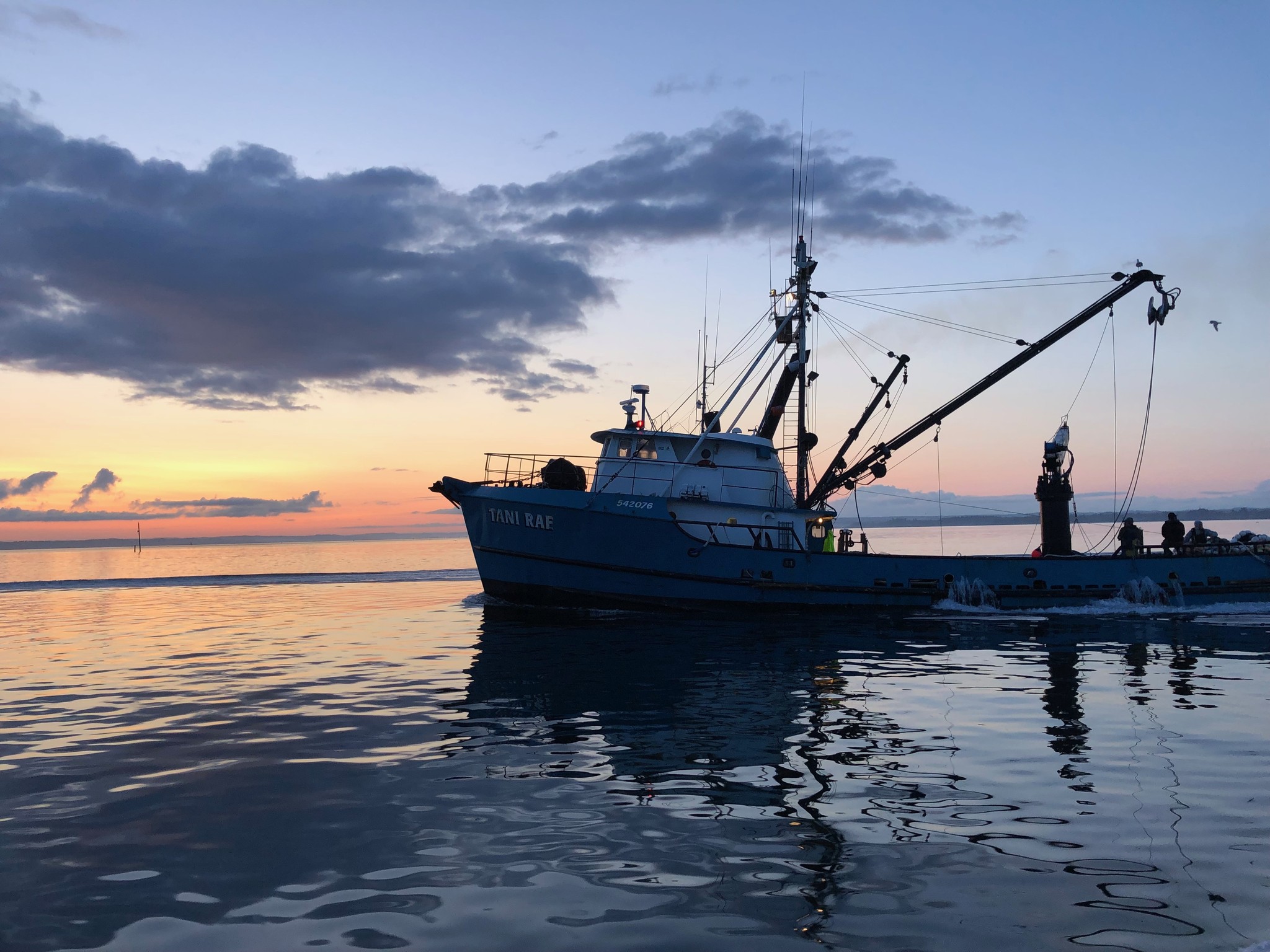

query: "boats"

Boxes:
[431, 96, 1270, 623]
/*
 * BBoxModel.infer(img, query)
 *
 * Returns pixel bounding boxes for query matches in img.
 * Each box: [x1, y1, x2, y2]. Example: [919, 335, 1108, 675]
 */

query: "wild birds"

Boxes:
[1209, 321, 1222, 331]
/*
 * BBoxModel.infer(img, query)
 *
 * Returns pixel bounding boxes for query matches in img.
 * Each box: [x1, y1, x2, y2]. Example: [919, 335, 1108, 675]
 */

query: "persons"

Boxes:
[1162, 512, 1218, 557]
[1117, 518, 1143, 559]
